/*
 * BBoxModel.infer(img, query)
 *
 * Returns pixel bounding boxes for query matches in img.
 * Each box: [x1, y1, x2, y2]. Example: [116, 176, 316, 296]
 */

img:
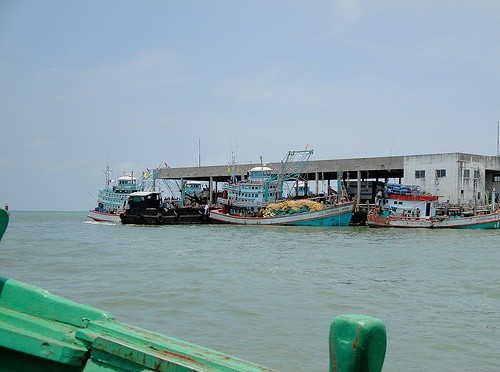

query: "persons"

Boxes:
[249, 207, 269, 217]
[415, 208, 420, 220]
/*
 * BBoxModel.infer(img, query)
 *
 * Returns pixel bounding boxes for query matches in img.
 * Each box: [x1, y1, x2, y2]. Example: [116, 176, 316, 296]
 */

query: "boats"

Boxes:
[361, 184, 500, 230]
[82, 161, 215, 225]
[209, 151, 359, 227]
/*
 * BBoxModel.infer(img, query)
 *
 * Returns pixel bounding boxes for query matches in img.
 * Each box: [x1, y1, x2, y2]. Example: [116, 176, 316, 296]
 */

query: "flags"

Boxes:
[227, 163, 230, 173]
[144, 169, 149, 178]
